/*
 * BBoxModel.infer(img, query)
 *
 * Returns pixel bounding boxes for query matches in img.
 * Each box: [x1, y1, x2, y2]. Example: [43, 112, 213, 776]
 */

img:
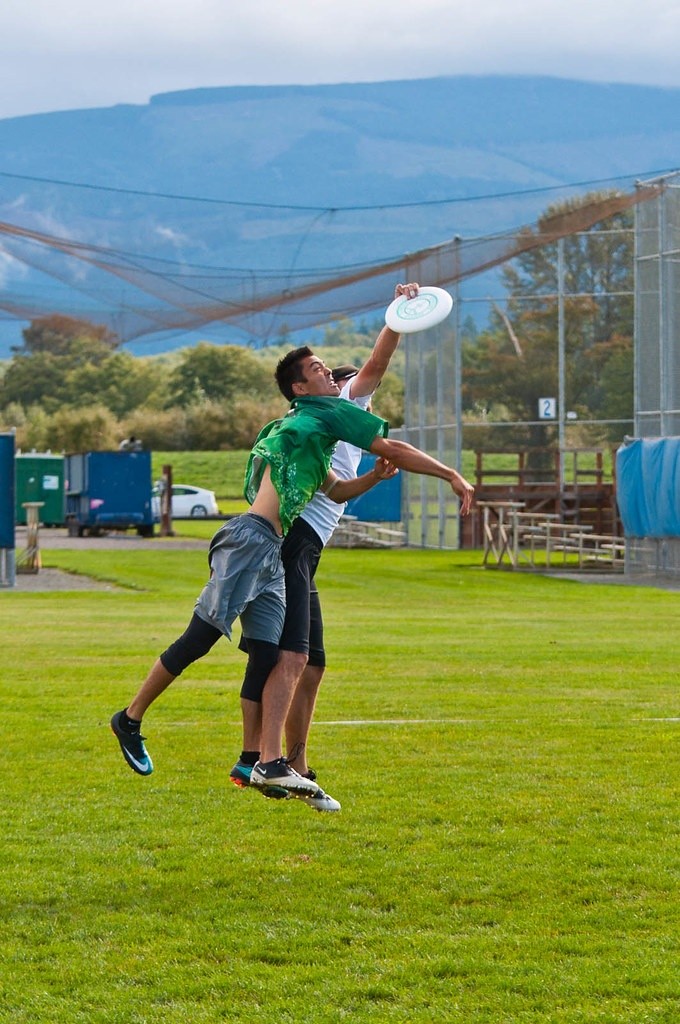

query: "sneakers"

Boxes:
[249, 761, 319, 793]
[230, 760, 289, 799]
[111, 712, 153, 776]
[284, 768, 341, 811]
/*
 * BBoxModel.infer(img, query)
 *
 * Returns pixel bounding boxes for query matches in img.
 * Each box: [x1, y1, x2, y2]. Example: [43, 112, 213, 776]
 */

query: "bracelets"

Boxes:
[324, 476, 341, 496]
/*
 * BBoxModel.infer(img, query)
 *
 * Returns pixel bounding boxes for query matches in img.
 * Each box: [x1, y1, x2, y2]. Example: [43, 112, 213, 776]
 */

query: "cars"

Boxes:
[152, 484, 220, 520]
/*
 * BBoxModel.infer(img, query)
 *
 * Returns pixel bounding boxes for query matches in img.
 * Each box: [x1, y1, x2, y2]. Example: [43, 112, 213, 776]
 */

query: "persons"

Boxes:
[111, 346, 475, 800]
[250, 283, 419, 813]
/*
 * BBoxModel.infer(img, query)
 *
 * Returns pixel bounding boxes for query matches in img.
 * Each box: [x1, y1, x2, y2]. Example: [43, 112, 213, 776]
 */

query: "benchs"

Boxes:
[325, 514, 408, 547]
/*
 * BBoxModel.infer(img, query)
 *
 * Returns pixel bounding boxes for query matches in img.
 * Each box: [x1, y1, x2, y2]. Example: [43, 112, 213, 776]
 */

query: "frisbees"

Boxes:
[384, 286, 453, 335]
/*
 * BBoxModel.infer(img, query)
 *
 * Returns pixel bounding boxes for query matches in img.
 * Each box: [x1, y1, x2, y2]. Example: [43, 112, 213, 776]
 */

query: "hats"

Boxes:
[332, 365, 381, 388]
